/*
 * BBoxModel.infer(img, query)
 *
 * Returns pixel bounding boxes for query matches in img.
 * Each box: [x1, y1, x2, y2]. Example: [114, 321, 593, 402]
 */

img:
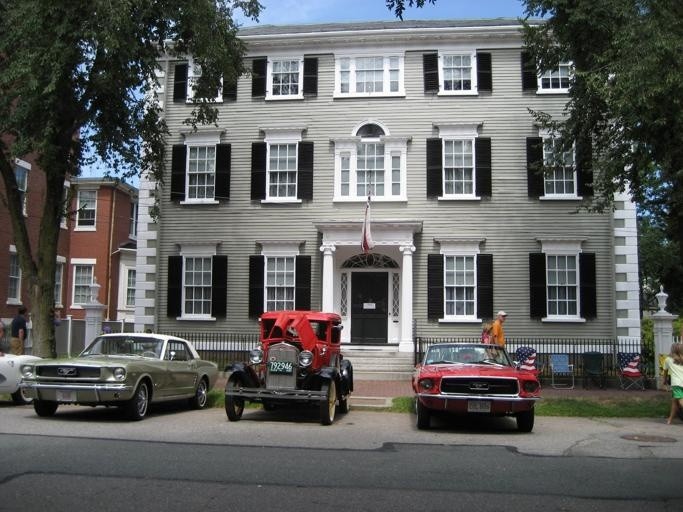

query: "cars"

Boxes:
[18, 330, 223, 421]
[0, 349, 44, 406]
[407, 341, 543, 436]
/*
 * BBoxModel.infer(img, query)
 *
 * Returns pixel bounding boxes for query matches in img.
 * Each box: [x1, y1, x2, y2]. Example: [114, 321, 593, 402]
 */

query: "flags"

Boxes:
[361, 190, 375, 255]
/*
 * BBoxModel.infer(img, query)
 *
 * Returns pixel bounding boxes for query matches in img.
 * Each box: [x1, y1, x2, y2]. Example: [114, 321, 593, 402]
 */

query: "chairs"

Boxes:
[580, 351, 608, 389]
[512, 346, 545, 386]
[616, 352, 647, 391]
[547, 353, 575, 390]
[657, 352, 673, 393]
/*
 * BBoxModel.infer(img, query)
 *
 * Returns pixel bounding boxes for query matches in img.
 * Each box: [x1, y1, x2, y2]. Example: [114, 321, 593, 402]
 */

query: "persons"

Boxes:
[492, 311, 508, 345]
[663, 343, 683, 424]
[11, 306, 28, 355]
[481, 323, 493, 344]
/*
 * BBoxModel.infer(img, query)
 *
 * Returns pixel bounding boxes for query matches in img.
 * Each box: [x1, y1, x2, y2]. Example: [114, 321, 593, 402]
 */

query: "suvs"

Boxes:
[222, 307, 357, 431]
